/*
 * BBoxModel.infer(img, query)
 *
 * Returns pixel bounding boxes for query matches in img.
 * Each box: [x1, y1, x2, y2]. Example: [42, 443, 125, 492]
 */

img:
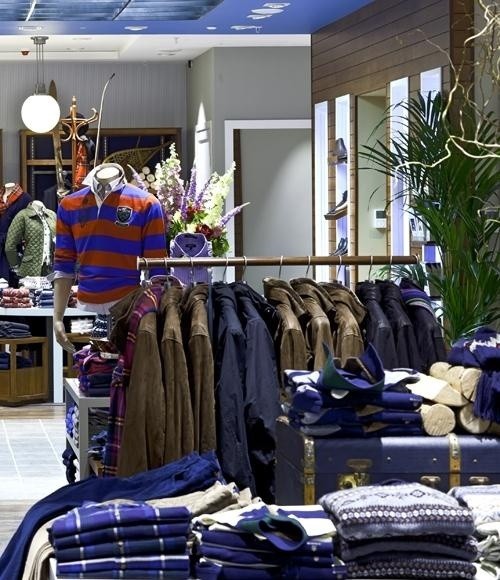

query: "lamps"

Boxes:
[21, 35, 61, 134]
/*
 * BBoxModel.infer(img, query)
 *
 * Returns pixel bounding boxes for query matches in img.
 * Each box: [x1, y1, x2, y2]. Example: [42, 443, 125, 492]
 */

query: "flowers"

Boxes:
[127, 140, 251, 256]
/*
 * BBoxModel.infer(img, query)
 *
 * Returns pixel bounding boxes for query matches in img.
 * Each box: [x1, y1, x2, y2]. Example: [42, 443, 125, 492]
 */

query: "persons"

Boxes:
[5, 199, 60, 279]
[0, 182, 30, 286]
[53, 162, 165, 353]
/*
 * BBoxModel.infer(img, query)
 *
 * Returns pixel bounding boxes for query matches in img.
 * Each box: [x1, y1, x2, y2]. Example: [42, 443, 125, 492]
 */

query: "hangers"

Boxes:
[139, 254, 421, 291]
